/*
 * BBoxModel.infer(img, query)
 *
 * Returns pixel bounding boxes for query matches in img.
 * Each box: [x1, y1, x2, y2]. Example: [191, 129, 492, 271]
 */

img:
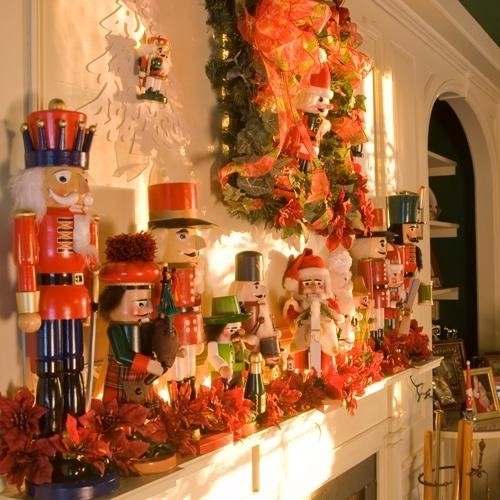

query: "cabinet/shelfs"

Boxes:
[428, 147, 462, 303]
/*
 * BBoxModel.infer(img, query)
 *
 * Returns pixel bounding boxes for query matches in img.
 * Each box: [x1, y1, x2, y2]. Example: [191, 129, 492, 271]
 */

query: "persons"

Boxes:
[472, 376, 495, 412]
[15, 100, 424, 499]
[293, 66, 335, 175]
[13, 98, 425, 500]
[136, 36, 170, 104]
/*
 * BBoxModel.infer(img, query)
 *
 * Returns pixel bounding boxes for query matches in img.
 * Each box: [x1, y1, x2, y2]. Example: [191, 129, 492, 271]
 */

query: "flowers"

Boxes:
[0, 379, 260, 498]
[206, 0, 375, 250]
[249, 319, 432, 425]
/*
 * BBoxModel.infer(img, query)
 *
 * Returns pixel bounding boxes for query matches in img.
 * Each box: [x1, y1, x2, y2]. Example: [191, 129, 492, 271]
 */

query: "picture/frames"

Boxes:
[432, 339, 500, 423]
